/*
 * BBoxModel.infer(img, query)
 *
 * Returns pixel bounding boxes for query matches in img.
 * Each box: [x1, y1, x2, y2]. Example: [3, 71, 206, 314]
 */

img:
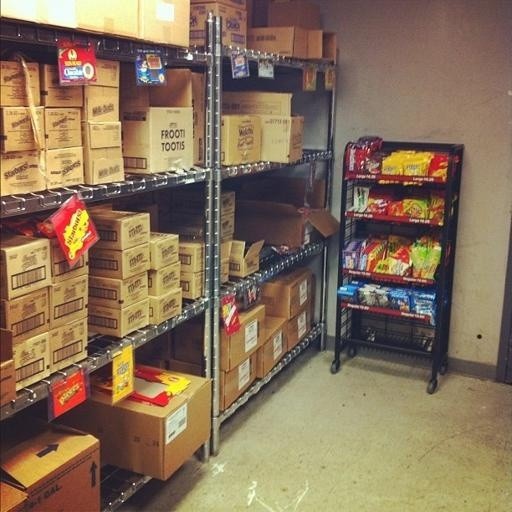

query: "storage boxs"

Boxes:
[0, 0, 337, 512]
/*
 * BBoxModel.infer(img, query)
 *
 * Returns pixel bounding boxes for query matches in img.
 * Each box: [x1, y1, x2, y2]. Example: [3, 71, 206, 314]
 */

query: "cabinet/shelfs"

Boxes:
[330, 141, 464, 394]
